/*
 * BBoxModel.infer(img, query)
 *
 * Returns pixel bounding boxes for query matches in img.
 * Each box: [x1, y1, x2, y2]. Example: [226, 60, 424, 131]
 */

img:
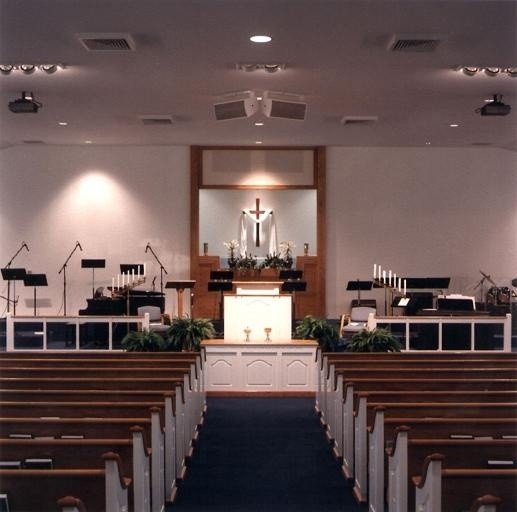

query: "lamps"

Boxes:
[240, 65, 258, 72]
[263, 64, 279, 73]
[462, 66, 517, 77]
[0, 62, 57, 75]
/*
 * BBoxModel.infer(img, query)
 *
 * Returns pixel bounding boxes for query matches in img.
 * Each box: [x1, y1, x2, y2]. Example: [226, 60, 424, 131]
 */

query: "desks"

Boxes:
[200, 339, 319, 392]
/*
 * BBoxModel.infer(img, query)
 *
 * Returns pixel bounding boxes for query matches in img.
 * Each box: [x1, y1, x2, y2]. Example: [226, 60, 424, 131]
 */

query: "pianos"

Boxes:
[79, 287, 165, 316]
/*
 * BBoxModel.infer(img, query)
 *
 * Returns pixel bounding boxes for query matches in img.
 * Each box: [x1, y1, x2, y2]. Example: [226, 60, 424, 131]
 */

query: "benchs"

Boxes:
[315, 349, 517, 512]
[0, 351, 207, 512]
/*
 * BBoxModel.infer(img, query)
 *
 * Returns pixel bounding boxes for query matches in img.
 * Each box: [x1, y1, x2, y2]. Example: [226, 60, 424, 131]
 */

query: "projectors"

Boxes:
[8, 98, 37, 112]
[480, 103, 511, 116]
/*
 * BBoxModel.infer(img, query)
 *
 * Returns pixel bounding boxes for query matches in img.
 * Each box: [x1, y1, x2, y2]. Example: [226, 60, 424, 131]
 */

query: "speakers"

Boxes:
[214, 94, 257, 121]
[266, 98, 308, 122]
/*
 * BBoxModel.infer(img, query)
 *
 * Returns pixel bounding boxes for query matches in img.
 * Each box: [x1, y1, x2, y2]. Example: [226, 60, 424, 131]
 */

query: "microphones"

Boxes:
[22, 240, 30, 252]
[144, 241, 150, 253]
[76, 240, 83, 251]
